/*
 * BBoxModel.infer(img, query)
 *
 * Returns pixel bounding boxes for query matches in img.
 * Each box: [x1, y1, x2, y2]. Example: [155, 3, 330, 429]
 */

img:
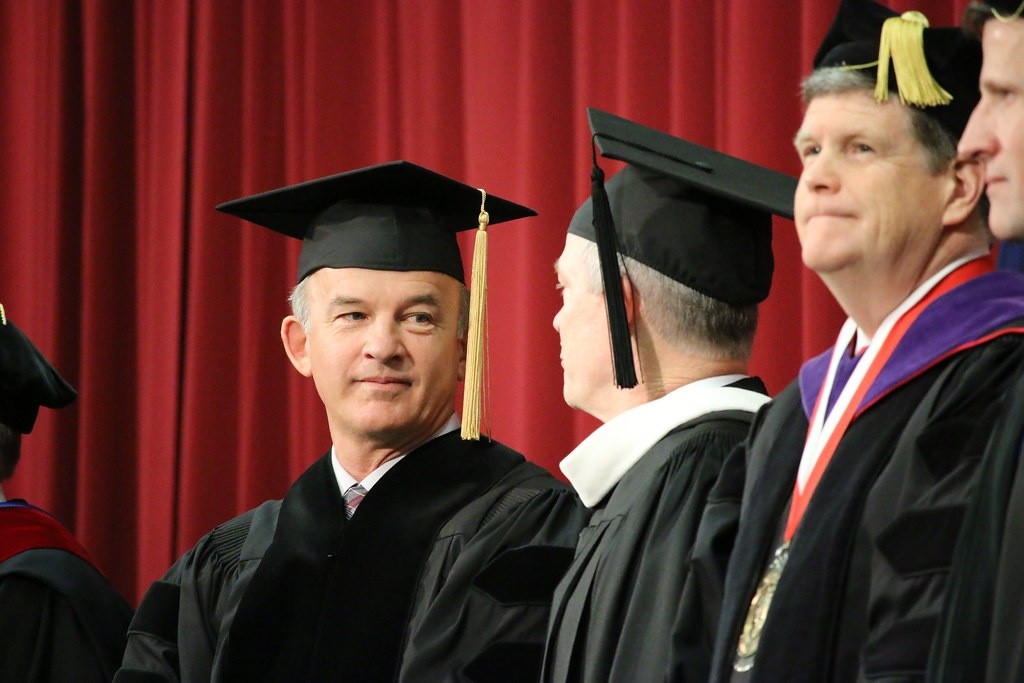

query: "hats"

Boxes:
[565, 107, 800, 389]
[812, 0, 982, 139]
[215, 160, 538, 441]
[0, 303, 80, 444]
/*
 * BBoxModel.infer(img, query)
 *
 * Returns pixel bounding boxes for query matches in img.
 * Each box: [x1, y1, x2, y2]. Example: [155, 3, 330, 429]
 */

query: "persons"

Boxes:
[116, 160, 554, 683]
[928, 0, 1024, 683]
[711, 0, 1024, 683]
[0, 304, 135, 683]
[540, 106, 801, 683]
